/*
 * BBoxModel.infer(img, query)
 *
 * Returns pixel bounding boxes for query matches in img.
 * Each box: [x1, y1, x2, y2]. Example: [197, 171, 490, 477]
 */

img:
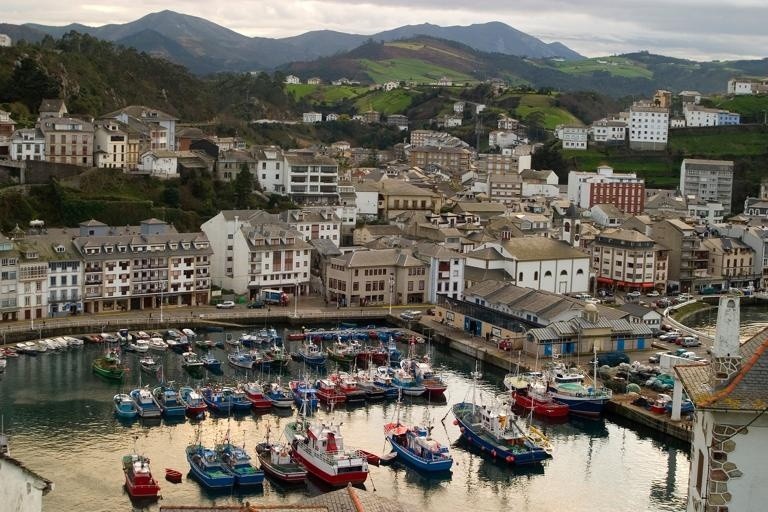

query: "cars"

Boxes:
[246, 300, 265, 309]
[576, 285, 702, 363]
[741, 287, 751, 292]
[0, 335, 86, 372]
[214, 300, 235, 310]
[426, 307, 436, 316]
[399, 308, 423, 321]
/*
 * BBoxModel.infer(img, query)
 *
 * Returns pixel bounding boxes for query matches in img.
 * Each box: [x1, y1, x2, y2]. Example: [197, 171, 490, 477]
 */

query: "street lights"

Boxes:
[624, 364, 636, 406]
[159, 279, 165, 322]
[293, 277, 300, 317]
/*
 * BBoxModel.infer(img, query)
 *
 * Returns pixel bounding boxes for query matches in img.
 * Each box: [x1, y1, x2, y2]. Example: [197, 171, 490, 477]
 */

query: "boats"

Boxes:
[185, 422, 237, 489]
[182, 346, 204, 372]
[177, 386, 208, 412]
[385, 386, 454, 477]
[503, 339, 609, 421]
[92, 345, 129, 380]
[121, 453, 162, 501]
[284, 382, 372, 487]
[200, 377, 234, 413]
[200, 350, 223, 371]
[96, 325, 224, 354]
[221, 322, 448, 412]
[649, 392, 695, 419]
[254, 419, 309, 482]
[113, 388, 138, 419]
[451, 359, 553, 468]
[139, 355, 162, 375]
[164, 467, 183, 482]
[130, 371, 163, 420]
[213, 417, 266, 486]
[152, 373, 187, 417]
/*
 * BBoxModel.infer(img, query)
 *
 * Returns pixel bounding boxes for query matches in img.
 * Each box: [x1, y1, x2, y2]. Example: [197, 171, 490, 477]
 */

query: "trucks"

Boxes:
[259, 288, 288, 306]
